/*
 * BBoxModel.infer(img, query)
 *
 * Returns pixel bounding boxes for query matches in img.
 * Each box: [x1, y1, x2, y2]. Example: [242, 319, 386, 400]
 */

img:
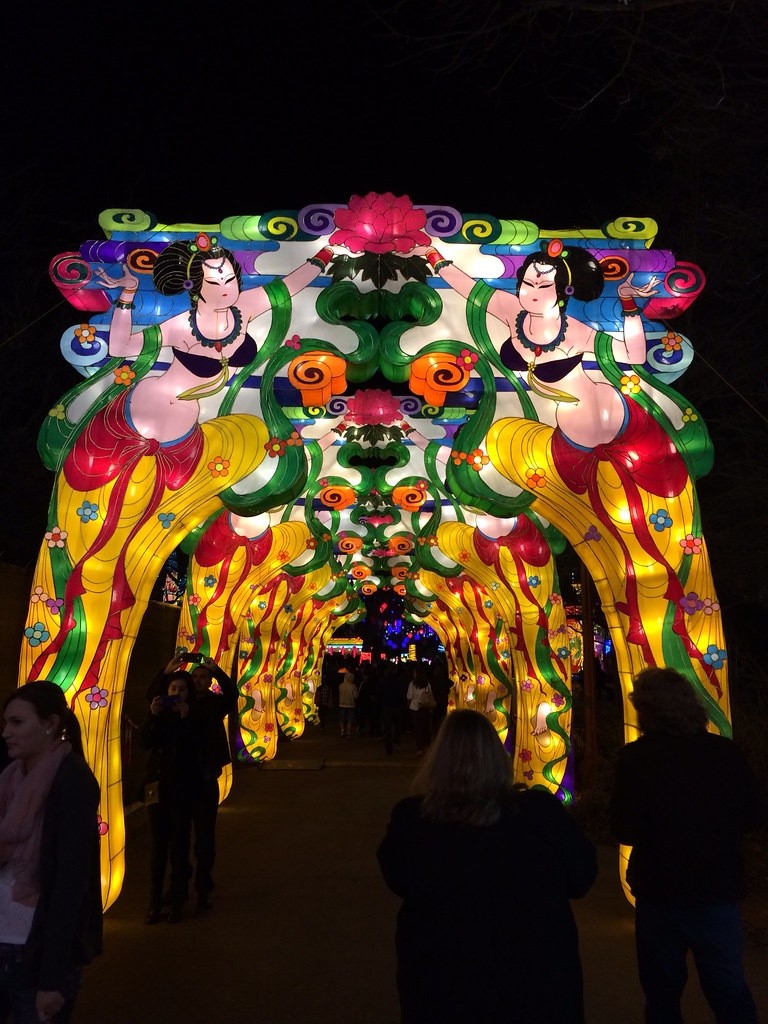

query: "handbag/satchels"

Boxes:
[421, 685, 437, 708]
[144, 781, 162, 808]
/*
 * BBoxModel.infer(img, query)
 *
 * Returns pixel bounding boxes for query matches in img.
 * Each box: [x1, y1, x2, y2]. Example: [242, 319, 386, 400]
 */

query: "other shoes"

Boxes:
[145, 908, 160, 924]
[163, 893, 171, 905]
[347, 732, 351, 737]
[199, 891, 214, 910]
[341, 729, 345, 736]
[168, 907, 182, 924]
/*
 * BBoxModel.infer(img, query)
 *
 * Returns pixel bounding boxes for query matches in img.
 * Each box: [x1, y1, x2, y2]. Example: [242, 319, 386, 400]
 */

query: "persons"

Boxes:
[609, 667, 768, 1024]
[139, 652, 240, 924]
[314, 649, 451, 760]
[374, 711, 600, 1024]
[0, 681, 104, 1024]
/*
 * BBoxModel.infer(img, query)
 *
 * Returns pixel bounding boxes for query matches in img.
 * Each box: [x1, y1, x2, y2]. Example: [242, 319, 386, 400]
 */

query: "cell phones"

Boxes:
[181, 653, 202, 662]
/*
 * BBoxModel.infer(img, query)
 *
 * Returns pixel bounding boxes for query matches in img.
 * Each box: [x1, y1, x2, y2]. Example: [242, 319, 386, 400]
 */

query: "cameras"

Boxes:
[159, 695, 180, 707]
